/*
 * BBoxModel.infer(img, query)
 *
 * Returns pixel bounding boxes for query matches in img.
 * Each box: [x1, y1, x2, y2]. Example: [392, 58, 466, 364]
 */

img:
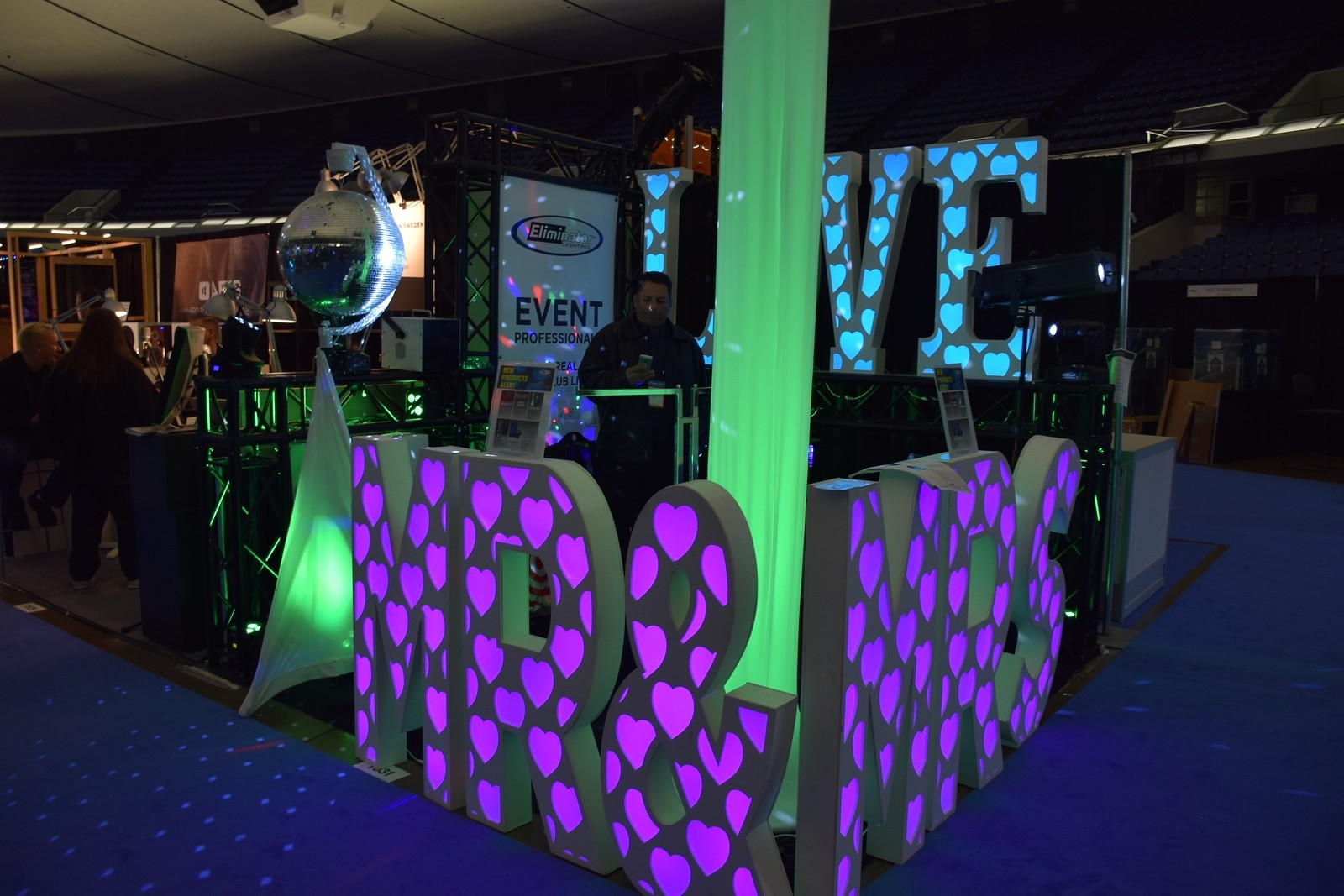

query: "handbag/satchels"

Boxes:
[545, 432, 598, 465]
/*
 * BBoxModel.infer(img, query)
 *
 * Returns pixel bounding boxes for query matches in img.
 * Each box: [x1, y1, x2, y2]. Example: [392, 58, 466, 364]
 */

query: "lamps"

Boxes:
[51, 287, 128, 352]
[260, 280, 313, 324]
[315, 141, 426, 208]
[204, 284, 267, 323]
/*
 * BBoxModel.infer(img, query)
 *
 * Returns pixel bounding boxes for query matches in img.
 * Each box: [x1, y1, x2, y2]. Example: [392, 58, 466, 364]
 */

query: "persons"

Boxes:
[75, 285, 105, 323]
[0, 308, 160, 590]
[579, 271, 708, 577]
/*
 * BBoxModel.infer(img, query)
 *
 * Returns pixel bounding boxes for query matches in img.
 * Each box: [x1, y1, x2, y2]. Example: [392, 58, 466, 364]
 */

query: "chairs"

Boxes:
[1132, 214, 1344, 281]
[825, 56, 1298, 152]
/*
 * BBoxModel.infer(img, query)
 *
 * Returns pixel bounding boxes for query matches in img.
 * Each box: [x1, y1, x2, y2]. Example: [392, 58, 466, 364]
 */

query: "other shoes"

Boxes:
[73, 578, 96, 590]
[120, 575, 140, 589]
[8, 499, 30, 530]
[27, 489, 58, 526]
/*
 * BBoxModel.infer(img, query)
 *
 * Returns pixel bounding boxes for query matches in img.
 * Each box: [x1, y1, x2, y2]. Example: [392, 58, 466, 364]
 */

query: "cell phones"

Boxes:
[639, 356, 652, 370]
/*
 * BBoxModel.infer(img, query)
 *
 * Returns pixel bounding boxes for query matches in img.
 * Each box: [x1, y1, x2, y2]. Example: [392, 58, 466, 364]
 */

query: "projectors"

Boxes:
[980, 251, 1117, 306]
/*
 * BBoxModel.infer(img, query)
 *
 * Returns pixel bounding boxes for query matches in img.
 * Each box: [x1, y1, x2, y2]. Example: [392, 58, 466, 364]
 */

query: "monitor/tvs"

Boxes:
[151, 326, 212, 435]
